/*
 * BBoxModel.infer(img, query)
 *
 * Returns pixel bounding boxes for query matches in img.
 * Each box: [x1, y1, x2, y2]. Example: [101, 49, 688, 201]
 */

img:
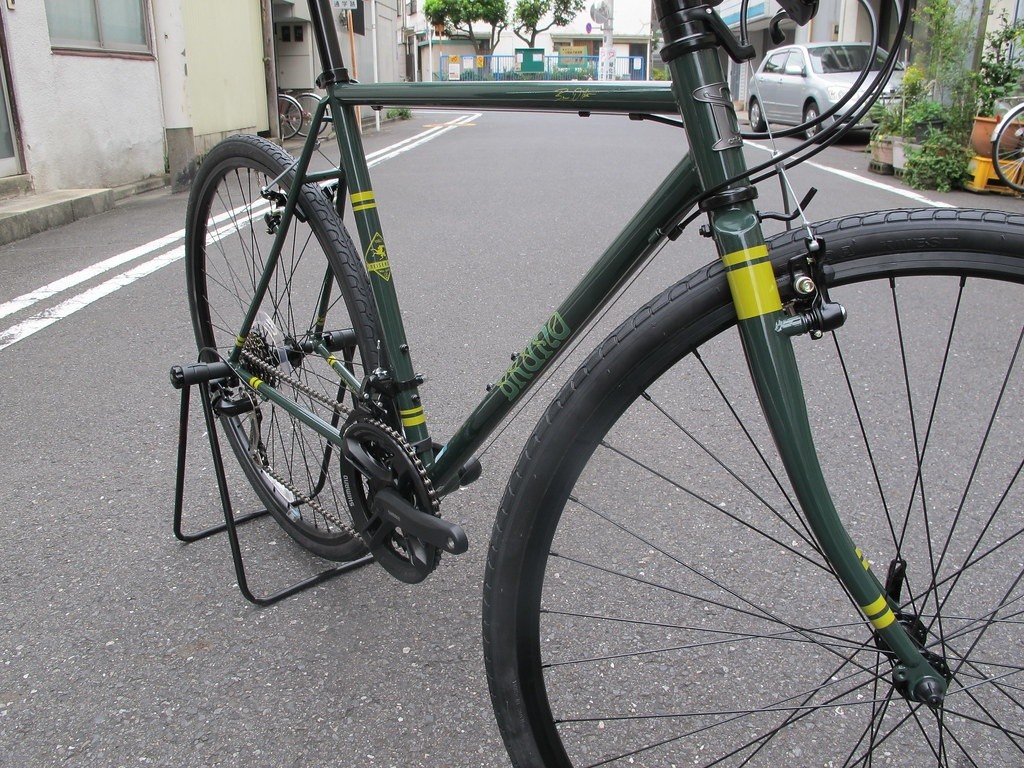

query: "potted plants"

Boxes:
[869, 0, 1023, 192]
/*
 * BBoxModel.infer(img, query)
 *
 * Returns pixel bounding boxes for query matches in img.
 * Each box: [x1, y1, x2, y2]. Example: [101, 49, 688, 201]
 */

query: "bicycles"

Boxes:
[168, 1, 1023, 768]
[989, 95, 1023, 193]
[274, 85, 330, 142]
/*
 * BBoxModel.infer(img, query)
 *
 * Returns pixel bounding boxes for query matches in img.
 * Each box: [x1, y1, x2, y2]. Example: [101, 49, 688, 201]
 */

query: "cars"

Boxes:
[745, 41, 924, 145]
